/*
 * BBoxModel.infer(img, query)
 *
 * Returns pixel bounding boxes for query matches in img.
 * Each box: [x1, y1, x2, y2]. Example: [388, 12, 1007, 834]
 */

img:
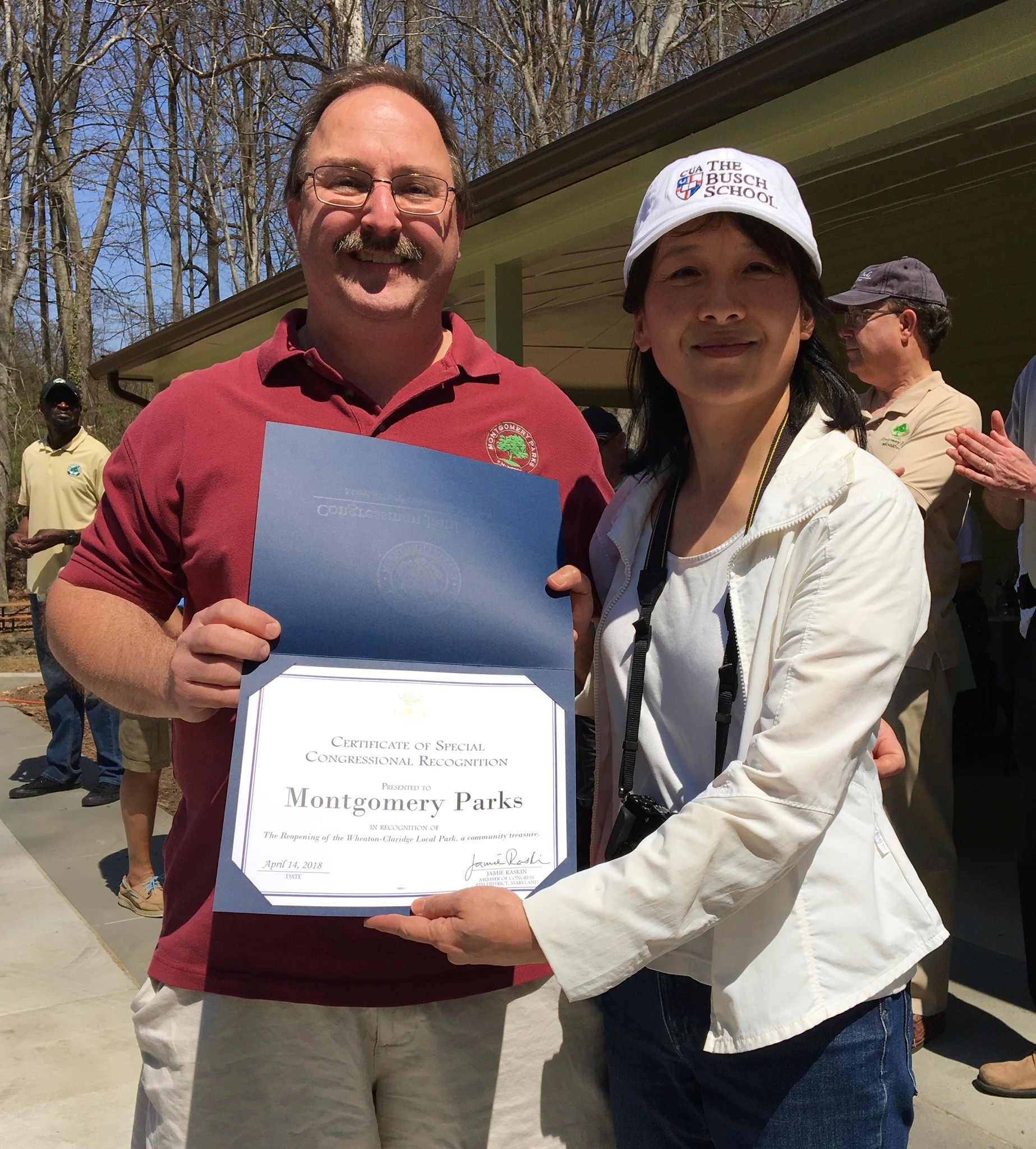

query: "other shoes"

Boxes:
[117, 873, 164, 918]
[976, 1049, 1036, 1098]
[911, 1011, 946, 1054]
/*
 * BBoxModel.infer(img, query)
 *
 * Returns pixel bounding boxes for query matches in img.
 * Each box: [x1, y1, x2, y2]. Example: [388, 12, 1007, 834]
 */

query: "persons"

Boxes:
[118, 596, 184, 918]
[46, 61, 905, 1149]
[577, 407, 635, 870]
[362, 146, 950, 1149]
[946, 354, 1036, 1098]
[8, 378, 123, 806]
[828, 256, 982, 1052]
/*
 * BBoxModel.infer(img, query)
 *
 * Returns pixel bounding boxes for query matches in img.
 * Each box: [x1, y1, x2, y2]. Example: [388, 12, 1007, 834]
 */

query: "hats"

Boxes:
[624, 148, 823, 285]
[823, 256, 947, 317]
[41, 378, 81, 401]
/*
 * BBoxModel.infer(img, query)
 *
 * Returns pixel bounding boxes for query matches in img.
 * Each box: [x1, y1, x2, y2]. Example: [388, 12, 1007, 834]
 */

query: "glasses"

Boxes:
[844, 308, 907, 330]
[296, 165, 460, 215]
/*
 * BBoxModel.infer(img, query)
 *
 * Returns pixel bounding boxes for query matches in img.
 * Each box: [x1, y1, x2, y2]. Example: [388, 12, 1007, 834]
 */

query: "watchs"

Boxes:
[65, 529, 78, 545]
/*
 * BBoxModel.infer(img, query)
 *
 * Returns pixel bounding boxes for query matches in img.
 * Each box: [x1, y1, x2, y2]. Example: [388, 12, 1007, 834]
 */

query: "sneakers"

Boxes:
[9, 776, 81, 799]
[81, 781, 120, 806]
